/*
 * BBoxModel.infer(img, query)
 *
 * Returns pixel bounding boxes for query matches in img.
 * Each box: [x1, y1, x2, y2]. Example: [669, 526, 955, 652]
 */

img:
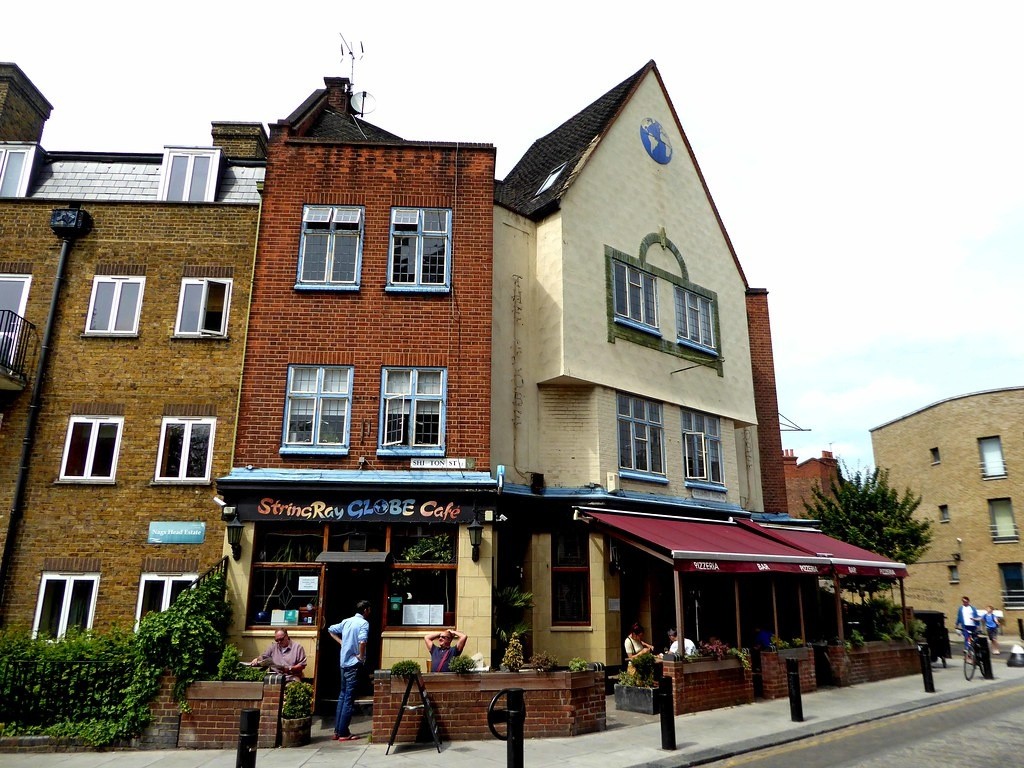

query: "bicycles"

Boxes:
[955, 626, 986, 681]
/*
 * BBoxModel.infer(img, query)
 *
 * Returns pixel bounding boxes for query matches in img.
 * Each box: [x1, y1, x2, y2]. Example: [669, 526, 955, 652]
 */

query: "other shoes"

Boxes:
[992, 649, 1000, 654]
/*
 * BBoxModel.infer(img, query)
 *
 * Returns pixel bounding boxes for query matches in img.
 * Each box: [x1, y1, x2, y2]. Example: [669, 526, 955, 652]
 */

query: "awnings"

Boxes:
[571, 505, 909, 577]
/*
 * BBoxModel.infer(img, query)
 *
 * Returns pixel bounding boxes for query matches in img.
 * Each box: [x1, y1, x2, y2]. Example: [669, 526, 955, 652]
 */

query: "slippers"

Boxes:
[332, 734, 360, 741]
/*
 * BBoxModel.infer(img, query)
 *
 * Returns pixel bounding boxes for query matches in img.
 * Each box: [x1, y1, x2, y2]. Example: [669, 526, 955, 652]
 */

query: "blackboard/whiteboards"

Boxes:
[387, 671, 442, 746]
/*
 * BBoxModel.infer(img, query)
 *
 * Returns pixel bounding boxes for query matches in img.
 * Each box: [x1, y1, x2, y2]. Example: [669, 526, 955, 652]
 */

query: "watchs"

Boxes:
[289, 666, 292, 672]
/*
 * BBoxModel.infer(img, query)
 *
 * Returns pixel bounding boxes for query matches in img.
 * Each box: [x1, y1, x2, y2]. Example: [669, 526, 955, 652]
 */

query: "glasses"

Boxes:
[438, 636, 449, 638]
[275, 634, 286, 642]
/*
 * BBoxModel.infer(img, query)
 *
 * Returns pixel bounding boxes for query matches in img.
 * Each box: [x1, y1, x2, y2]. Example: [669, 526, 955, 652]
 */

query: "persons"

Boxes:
[841, 604, 855, 639]
[657, 627, 697, 660]
[969, 606, 1000, 655]
[250, 628, 307, 683]
[423, 629, 467, 673]
[327, 599, 370, 742]
[953, 596, 980, 660]
[624, 623, 654, 676]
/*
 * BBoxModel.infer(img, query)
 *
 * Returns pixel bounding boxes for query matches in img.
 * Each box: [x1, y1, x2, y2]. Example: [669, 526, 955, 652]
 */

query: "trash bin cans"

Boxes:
[913, 609, 947, 668]
